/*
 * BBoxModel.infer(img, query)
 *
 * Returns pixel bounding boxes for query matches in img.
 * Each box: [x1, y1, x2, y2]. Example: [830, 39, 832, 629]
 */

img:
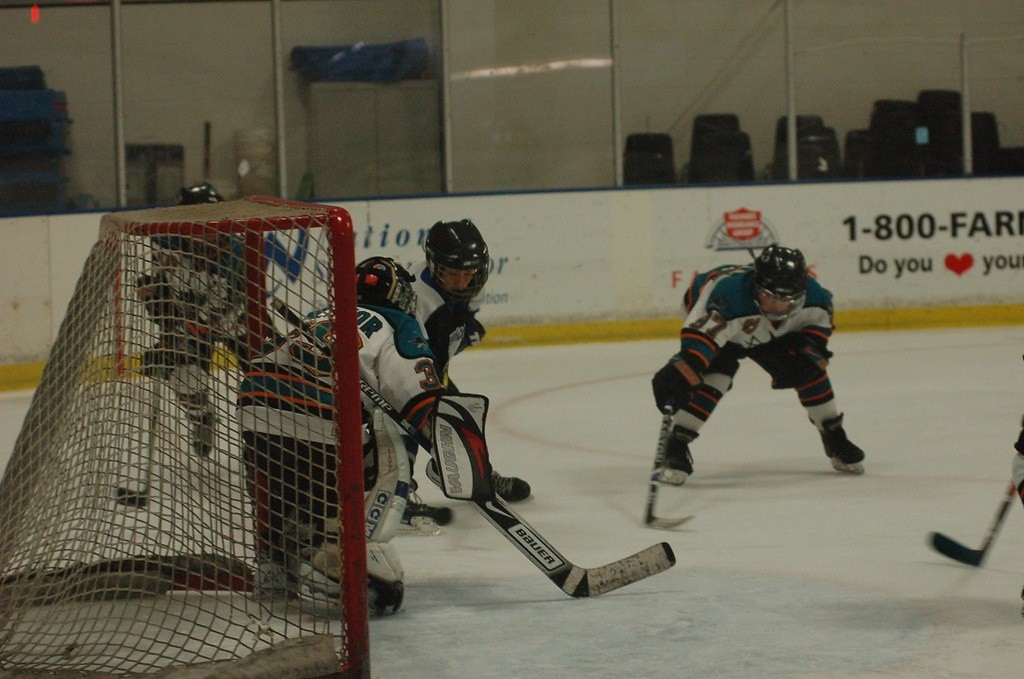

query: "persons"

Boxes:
[133, 178, 282, 461]
[648, 241, 868, 475]
[1007, 326, 1024, 621]
[233, 218, 532, 616]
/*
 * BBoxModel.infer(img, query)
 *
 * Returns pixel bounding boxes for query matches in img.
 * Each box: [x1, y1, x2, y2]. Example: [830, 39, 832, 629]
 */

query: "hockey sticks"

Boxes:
[639, 396, 699, 531]
[115, 376, 162, 509]
[443, 363, 451, 392]
[927, 480, 1018, 571]
[268, 295, 678, 601]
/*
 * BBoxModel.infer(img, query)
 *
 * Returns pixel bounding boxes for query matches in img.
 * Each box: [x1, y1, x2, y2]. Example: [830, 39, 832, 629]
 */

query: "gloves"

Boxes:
[652, 345, 709, 417]
[787, 325, 833, 388]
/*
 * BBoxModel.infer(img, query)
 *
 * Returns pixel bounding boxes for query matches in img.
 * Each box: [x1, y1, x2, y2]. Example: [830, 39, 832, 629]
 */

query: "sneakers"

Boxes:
[819, 413, 866, 473]
[397, 488, 452, 525]
[490, 470, 531, 500]
[176, 400, 217, 455]
[659, 424, 700, 484]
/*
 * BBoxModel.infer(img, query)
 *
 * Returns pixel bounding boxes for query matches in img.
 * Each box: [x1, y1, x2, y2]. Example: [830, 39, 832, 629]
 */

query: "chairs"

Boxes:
[621, 86, 1024, 188]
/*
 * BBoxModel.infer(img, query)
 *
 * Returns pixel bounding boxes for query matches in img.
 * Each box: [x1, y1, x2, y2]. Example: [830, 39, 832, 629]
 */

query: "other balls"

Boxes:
[435, 507, 454, 527]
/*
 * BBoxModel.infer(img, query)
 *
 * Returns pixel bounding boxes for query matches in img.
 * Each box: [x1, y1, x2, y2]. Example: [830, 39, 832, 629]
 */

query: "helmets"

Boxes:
[357, 256, 418, 320]
[752, 244, 808, 322]
[426, 219, 489, 307]
[177, 182, 234, 266]
